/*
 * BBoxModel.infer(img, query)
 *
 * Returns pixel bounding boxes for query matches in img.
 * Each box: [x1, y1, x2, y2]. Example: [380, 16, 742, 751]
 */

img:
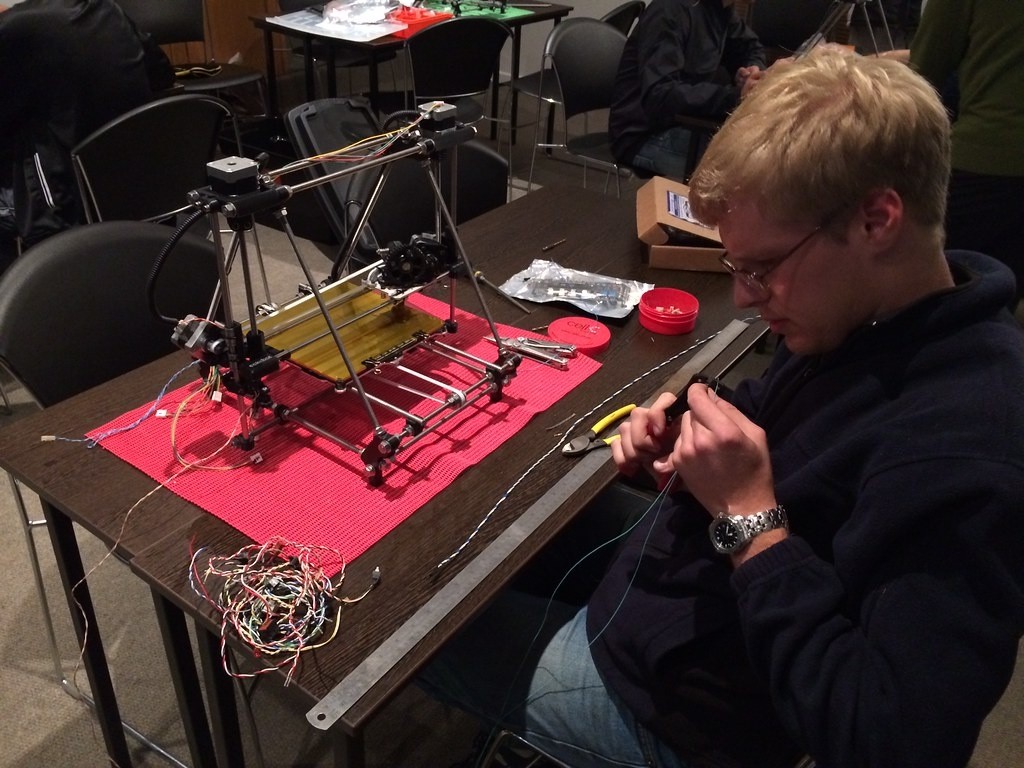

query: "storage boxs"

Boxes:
[635, 176, 730, 273]
[639, 287, 699, 336]
[386, 8, 453, 38]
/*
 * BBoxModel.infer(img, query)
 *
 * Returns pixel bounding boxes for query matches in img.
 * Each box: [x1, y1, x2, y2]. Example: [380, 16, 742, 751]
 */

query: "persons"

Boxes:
[411, 0, 1024, 768]
[0, 0, 176, 240]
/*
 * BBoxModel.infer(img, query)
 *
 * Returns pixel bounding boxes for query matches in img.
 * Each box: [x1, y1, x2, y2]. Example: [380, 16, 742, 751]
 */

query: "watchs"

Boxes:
[708, 505, 790, 558]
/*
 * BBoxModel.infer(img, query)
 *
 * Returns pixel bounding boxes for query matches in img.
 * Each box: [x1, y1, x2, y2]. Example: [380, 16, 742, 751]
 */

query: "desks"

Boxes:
[0, 180, 771, 767]
[246, 0, 575, 143]
[675, 41, 797, 185]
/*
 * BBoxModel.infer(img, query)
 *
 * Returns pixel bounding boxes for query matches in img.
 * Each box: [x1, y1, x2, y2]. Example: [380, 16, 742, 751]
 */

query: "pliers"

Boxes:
[483, 334, 578, 370]
[557, 403, 639, 455]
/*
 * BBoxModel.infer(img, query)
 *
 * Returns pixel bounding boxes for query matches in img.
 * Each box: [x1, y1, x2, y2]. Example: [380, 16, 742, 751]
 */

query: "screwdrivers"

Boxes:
[460, 263, 533, 316]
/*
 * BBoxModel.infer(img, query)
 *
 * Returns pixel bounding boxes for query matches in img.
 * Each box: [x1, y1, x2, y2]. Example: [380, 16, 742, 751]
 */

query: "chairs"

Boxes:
[0, 0, 648, 768]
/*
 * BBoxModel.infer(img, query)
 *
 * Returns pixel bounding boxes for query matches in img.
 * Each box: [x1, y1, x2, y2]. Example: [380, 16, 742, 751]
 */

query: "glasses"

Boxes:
[717, 225, 827, 294]
